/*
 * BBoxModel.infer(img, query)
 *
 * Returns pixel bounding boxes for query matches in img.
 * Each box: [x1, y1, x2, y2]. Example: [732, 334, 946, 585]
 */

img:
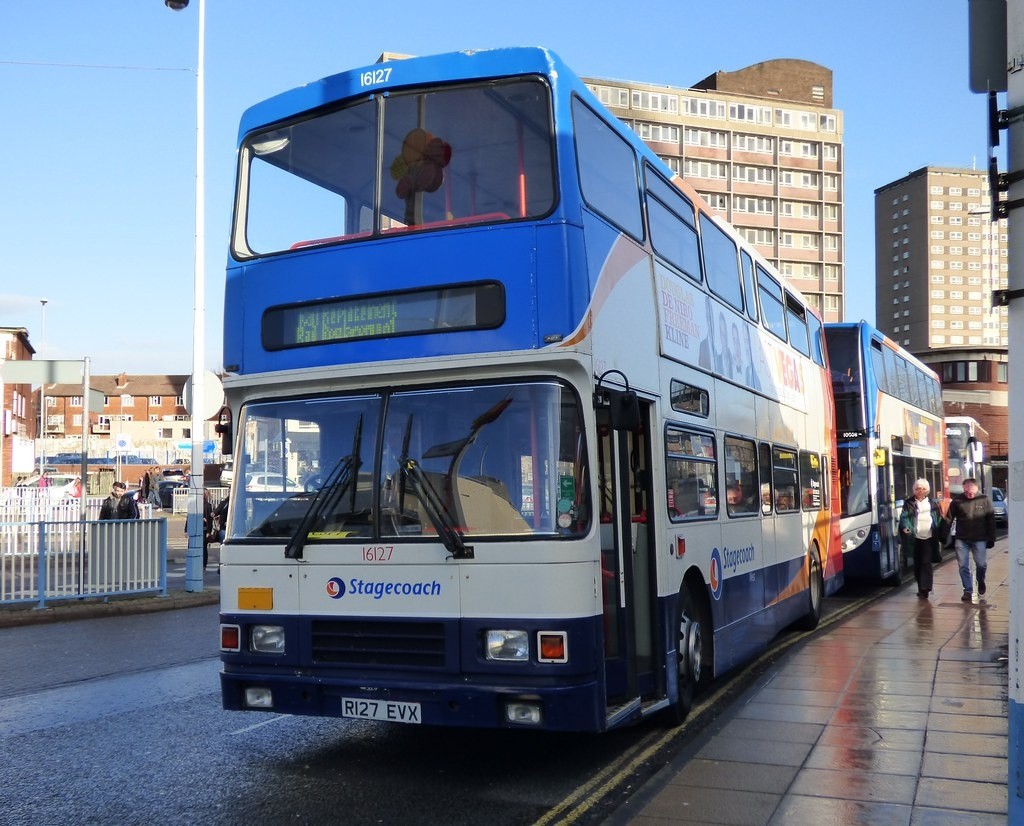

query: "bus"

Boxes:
[820, 320, 953, 588]
[218, 49, 842, 735]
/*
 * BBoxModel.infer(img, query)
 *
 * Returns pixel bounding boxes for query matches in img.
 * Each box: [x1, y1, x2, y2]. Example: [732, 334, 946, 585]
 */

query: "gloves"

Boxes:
[939, 537, 947, 545]
[986, 540, 994, 550]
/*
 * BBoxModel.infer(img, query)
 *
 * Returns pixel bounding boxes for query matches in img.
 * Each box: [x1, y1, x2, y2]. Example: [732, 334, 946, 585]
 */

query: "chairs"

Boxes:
[668, 478, 703, 517]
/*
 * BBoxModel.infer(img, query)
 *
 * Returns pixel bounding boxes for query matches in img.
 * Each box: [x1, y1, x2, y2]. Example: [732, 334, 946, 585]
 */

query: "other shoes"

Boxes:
[962, 592, 972, 600]
[156, 508, 162, 512]
[203, 567, 206, 575]
[917, 587, 928, 599]
[978, 582, 986, 595]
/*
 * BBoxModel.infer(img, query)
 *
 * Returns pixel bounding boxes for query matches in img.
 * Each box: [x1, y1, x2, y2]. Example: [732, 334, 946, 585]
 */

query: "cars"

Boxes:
[945, 416, 993, 550]
[993, 487, 1008, 525]
[244, 472, 304, 494]
[219, 459, 254, 487]
[161, 469, 186, 484]
[16, 472, 81, 500]
[125, 481, 188, 508]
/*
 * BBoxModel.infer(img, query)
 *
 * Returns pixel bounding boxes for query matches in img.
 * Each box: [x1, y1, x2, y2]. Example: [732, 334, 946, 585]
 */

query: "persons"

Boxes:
[184, 487, 212, 575]
[727, 480, 749, 514]
[142, 467, 163, 513]
[900, 478, 944, 598]
[181, 470, 192, 487]
[698, 296, 762, 392]
[944, 479, 996, 600]
[100, 482, 142, 520]
[211, 490, 230, 573]
[38, 472, 52, 487]
[761, 483, 779, 513]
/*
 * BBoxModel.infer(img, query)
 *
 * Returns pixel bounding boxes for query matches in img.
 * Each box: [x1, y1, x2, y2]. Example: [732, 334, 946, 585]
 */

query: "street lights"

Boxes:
[38, 298, 48, 471]
[163, 0, 204, 595]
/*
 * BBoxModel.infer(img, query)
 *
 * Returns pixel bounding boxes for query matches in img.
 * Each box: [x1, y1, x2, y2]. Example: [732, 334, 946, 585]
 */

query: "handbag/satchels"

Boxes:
[213, 529, 221, 541]
[930, 541, 943, 562]
[206, 533, 214, 543]
